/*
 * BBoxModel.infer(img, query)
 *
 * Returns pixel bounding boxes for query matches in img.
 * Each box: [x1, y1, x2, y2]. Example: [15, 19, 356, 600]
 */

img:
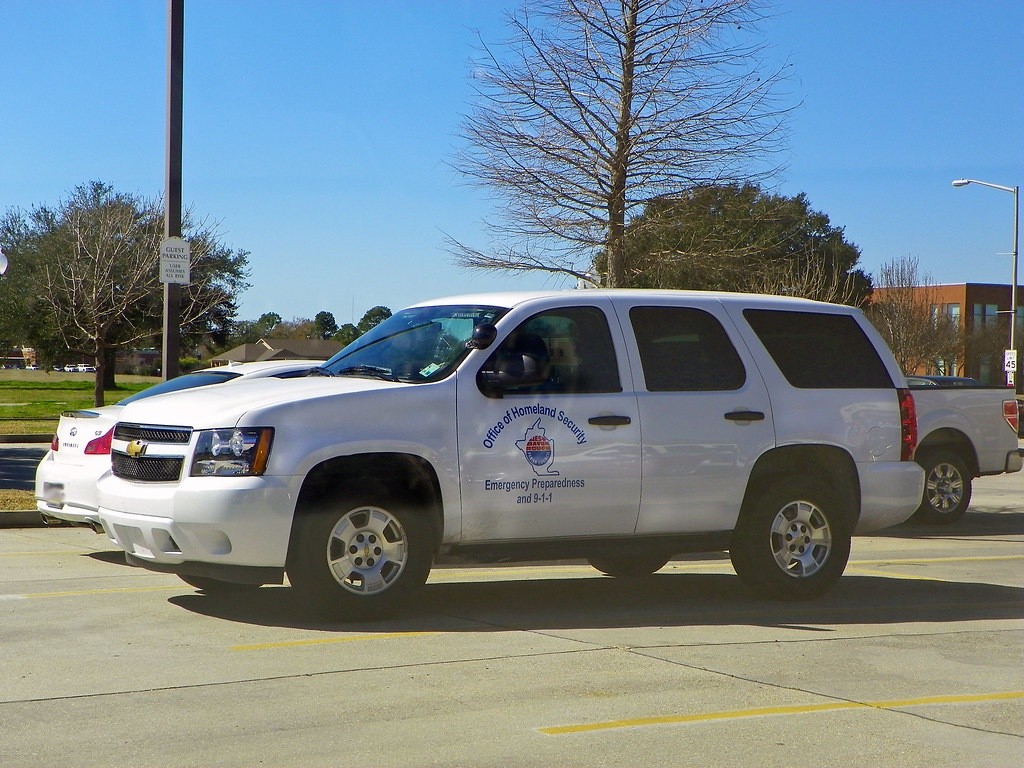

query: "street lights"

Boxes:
[951, 175, 1019, 384]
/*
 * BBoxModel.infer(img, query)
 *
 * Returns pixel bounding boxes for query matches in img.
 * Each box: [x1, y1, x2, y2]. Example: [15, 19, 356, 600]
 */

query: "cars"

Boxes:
[28, 353, 390, 535]
[26, 364, 39, 370]
[906, 374, 981, 387]
[53, 365, 64, 371]
[3, 364, 13, 369]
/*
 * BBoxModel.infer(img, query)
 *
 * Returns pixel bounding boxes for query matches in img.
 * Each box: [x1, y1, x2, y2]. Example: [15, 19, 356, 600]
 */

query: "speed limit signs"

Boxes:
[1004, 349, 1017, 373]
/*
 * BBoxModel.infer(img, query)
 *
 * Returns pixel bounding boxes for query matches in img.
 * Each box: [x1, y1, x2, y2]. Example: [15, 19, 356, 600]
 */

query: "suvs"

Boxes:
[64, 365, 79, 372]
[92, 288, 944, 629]
[77, 363, 96, 372]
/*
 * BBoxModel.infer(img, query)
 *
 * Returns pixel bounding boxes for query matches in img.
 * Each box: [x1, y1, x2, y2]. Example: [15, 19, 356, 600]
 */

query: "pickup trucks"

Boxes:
[903, 383, 1024, 522]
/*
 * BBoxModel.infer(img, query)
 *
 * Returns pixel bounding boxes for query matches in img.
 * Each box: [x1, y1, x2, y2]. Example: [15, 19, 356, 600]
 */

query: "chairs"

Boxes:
[513, 334, 550, 382]
